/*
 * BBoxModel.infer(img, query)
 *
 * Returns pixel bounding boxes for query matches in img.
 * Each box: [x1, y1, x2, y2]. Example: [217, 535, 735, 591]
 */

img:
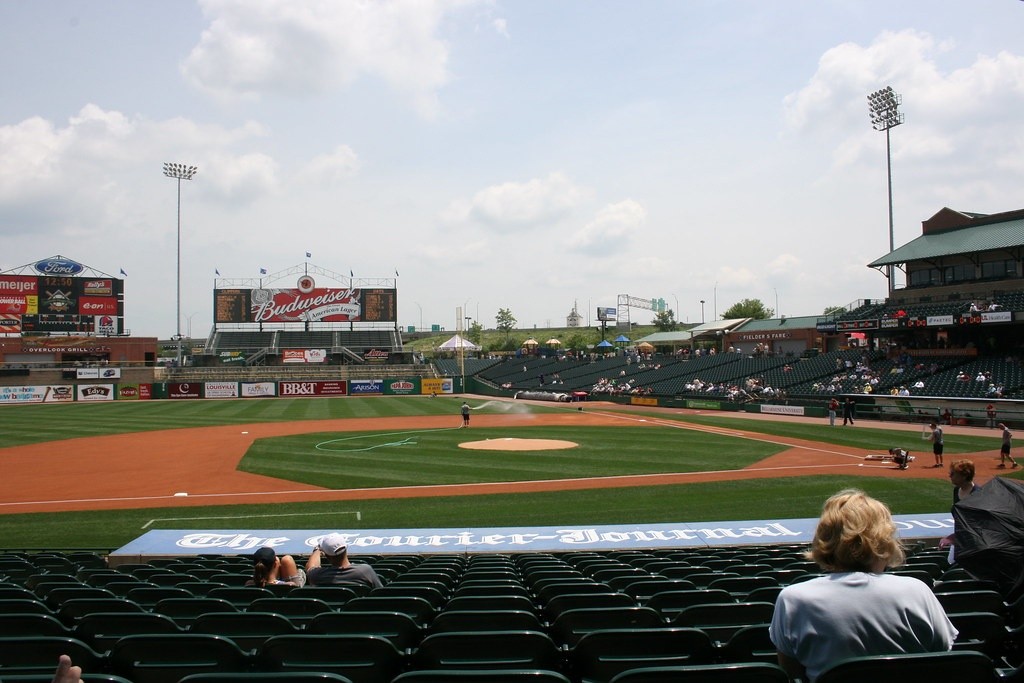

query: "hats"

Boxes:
[318, 533, 347, 556]
[253, 546, 276, 569]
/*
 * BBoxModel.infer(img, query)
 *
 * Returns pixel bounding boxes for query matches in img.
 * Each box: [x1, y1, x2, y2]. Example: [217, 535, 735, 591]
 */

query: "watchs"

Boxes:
[313, 547, 321, 552]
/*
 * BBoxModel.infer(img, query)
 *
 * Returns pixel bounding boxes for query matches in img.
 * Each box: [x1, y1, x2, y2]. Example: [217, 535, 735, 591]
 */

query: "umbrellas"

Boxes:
[437, 334, 481, 359]
[949, 468, 1024, 613]
[597, 340, 612, 353]
[638, 341, 654, 359]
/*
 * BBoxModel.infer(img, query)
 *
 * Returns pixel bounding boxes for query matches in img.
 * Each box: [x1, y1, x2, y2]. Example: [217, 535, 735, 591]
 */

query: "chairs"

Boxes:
[0, 543, 1024, 683]
[828, 290, 1024, 327]
[432, 348, 1024, 401]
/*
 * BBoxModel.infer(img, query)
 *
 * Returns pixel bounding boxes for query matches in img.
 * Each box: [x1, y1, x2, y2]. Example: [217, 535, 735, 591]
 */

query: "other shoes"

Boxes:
[1011, 463, 1019, 468]
[934, 463, 944, 467]
[997, 463, 1006, 467]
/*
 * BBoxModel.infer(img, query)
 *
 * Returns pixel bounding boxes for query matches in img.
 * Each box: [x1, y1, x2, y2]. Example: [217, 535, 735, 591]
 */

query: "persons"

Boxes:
[461, 401, 470, 428]
[938, 458, 983, 569]
[889, 448, 912, 468]
[997, 423, 1019, 469]
[925, 421, 944, 468]
[500, 299, 1023, 406]
[305, 533, 384, 588]
[768, 486, 961, 682]
[245, 547, 306, 588]
[829, 398, 839, 427]
[941, 408, 951, 425]
[986, 404, 996, 428]
[842, 398, 855, 426]
